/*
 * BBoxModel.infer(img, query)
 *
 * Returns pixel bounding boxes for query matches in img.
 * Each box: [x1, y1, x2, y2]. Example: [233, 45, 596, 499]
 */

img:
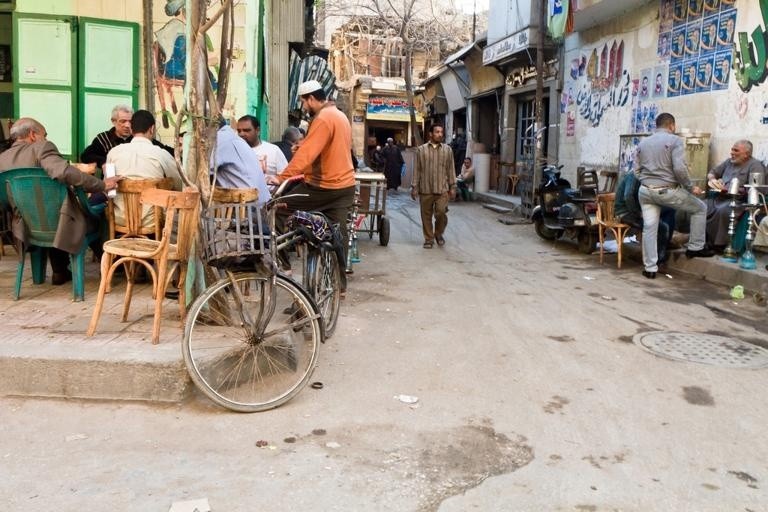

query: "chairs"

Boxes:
[501, 160, 520, 196]
[86, 187, 201, 344]
[596, 190, 644, 269]
[517, 161, 530, 195]
[0, 163, 261, 302]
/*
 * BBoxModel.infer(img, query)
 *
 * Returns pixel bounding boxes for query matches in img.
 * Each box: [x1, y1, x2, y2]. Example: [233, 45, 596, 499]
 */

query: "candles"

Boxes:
[748, 188, 760, 205]
[729, 177, 740, 194]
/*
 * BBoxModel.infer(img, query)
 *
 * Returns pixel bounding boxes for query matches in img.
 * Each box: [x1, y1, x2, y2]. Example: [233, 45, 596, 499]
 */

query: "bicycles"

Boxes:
[182, 174, 348, 414]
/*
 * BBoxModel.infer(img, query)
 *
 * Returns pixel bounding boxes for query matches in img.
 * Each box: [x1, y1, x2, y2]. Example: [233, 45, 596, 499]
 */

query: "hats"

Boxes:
[297, 79, 324, 97]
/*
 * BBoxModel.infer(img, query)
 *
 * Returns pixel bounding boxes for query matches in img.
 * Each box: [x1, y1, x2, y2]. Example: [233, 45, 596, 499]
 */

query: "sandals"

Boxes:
[435, 234, 446, 246]
[424, 241, 435, 250]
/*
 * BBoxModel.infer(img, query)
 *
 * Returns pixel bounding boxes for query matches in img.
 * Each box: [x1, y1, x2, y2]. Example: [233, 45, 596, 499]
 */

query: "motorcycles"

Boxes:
[531, 164, 602, 253]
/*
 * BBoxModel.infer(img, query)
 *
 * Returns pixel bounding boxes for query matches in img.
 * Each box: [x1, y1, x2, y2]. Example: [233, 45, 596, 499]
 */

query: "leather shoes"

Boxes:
[52, 268, 73, 285]
[686, 248, 715, 259]
[642, 269, 658, 279]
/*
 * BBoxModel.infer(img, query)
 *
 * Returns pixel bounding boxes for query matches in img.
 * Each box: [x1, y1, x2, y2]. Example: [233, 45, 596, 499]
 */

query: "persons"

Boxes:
[702, 139, 768, 255]
[0, 117, 125, 286]
[637, 1, 734, 96]
[79, 106, 166, 209]
[613, 168, 678, 262]
[271, 126, 301, 164]
[409, 123, 456, 248]
[266, 79, 358, 299]
[177, 130, 188, 160]
[286, 108, 303, 128]
[104, 109, 184, 300]
[567, 87, 574, 106]
[455, 157, 475, 201]
[236, 114, 291, 176]
[370, 145, 385, 172]
[380, 137, 404, 191]
[163, 108, 273, 300]
[632, 112, 714, 279]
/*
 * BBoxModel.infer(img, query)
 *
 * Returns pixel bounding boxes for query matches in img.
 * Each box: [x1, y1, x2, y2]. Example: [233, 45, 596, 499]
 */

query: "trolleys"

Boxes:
[348, 168, 391, 245]
